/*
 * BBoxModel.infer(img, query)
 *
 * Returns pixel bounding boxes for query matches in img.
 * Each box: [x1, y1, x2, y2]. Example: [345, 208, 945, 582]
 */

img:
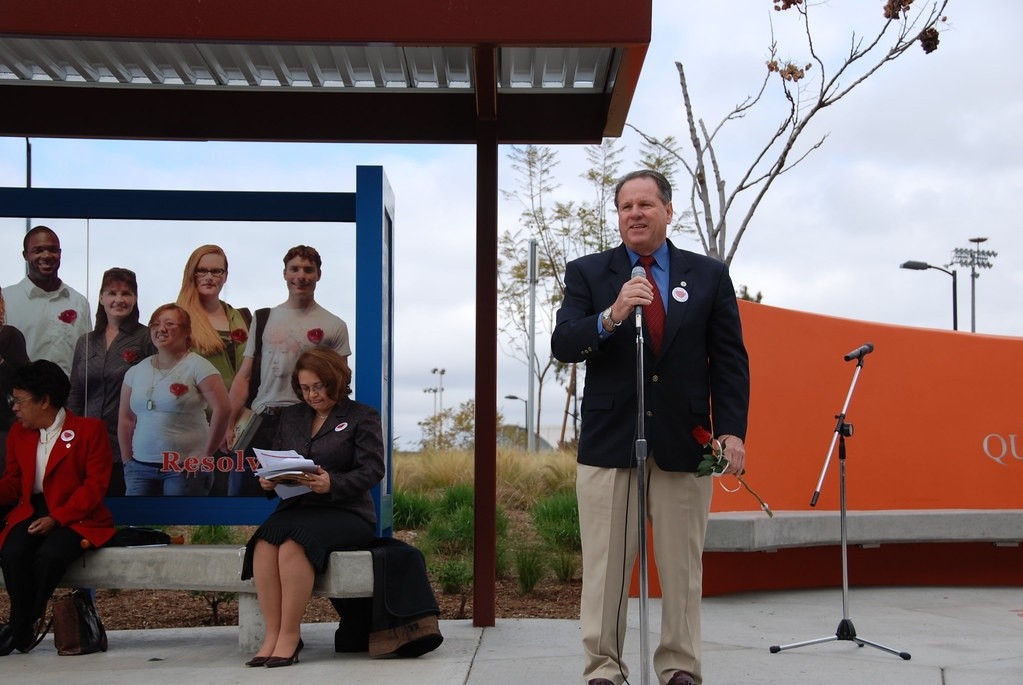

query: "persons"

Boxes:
[0, 354, 114, 656]
[551, 169, 750, 685]
[240, 348, 386, 668]
[1, 224, 351, 496]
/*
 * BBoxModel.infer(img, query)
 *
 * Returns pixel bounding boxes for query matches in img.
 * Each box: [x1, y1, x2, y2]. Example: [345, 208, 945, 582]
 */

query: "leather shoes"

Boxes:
[588, 677, 614, 685]
[666, 670, 698, 685]
[0, 622, 34, 655]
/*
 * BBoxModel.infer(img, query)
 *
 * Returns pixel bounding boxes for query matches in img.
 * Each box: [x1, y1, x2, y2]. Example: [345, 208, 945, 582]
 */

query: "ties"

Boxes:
[639, 255, 666, 363]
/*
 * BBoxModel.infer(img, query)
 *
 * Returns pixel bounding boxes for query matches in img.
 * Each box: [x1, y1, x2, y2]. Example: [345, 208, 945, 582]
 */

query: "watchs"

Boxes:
[603, 307, 623, 327]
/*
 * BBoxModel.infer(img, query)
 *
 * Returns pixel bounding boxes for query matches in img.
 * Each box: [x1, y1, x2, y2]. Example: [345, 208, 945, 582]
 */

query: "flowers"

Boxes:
[692, 425, 773, 520]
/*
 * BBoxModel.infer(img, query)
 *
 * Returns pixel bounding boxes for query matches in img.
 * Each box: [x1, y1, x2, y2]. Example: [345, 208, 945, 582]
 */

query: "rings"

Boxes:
[309, 485, 313, 489]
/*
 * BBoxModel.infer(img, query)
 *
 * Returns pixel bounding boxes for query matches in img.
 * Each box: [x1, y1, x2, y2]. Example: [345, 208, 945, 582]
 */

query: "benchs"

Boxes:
[696, 509, 1023, 552]
[0, 545, 374, 653]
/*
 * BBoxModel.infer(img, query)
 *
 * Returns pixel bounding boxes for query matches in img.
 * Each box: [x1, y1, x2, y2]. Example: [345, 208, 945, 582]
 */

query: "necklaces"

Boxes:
[39, 425, 62, 444]
[146, 348, 189, 411]
[315, 413, 327, 419]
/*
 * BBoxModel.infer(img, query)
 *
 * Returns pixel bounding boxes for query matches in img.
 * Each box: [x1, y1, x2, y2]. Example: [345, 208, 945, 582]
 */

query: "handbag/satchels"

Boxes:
[52, 588, 108, 656]
[243, 350, 261, 409]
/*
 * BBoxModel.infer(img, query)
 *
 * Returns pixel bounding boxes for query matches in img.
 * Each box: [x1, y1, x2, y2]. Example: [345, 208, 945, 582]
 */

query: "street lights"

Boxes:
[420, 366, 446, 457]
[952, 233, 996, 331]
[504, 393, 528, 450]
[899, 259, 958, 332]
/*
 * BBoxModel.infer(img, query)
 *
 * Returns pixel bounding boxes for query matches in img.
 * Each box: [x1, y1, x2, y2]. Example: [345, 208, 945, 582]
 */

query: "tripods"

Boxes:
[770, 358, 911, 660]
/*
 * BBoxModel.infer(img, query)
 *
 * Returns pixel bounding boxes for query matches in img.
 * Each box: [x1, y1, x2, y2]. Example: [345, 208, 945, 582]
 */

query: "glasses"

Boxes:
[194, 269, 225, 276]
[8, 393, 33, 408]
[148, 322, 182, 330]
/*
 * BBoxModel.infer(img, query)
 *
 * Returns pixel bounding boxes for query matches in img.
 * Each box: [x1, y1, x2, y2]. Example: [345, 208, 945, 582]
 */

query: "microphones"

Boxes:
[845, 343, 875, 361]
[632, 266, 647, 334]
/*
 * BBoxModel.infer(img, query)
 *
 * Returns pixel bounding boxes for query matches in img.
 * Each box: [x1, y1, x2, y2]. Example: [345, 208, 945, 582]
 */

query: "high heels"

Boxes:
[246, 637, 305, 667]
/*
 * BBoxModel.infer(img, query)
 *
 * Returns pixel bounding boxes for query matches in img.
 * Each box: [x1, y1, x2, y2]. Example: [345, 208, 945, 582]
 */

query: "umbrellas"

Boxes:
[81, 524, 186, 567]
